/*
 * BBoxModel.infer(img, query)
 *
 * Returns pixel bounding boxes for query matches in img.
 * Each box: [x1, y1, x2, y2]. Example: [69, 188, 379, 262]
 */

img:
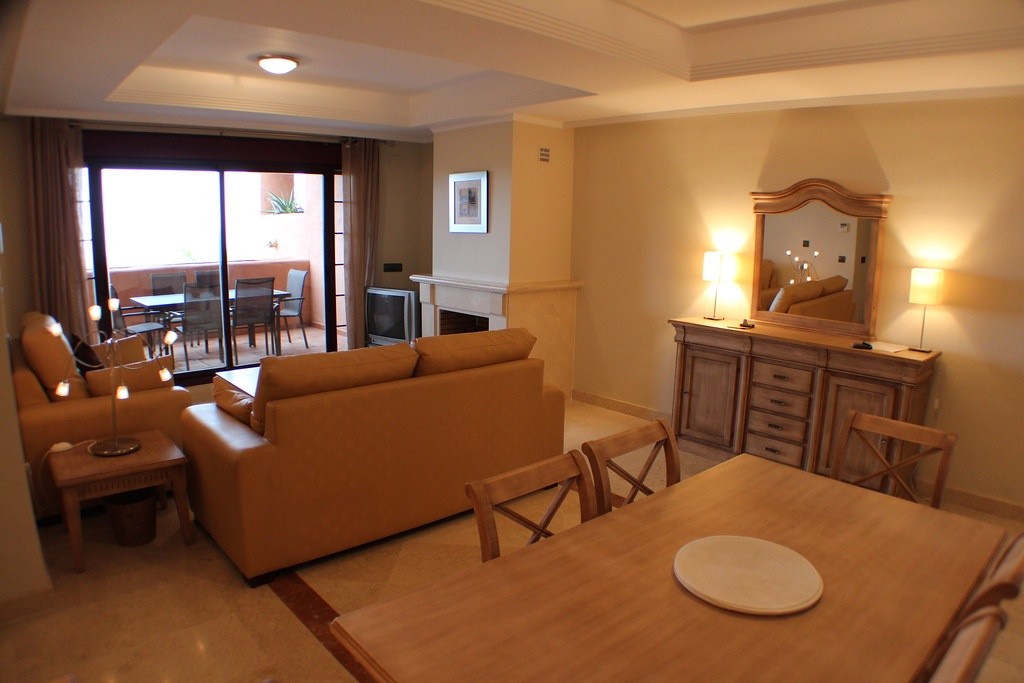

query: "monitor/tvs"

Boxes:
[365, 287, 421, 345]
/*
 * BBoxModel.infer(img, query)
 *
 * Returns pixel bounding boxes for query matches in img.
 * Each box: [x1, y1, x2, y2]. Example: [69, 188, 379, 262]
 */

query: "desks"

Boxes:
[48, 428, 196, 569]
[329, 453, 1002, 683]
[56, 299, 178, 458]
[129, 287, 291, 360]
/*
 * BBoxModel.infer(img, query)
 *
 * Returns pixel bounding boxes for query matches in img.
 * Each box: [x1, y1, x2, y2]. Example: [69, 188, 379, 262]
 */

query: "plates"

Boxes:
[673, 536, 823, 615]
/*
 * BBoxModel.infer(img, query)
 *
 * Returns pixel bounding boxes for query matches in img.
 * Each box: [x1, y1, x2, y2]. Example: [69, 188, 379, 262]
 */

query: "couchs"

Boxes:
[9, 312, 191, 529]
[178, 327, 566, 587]
[759, 260, 854, 323]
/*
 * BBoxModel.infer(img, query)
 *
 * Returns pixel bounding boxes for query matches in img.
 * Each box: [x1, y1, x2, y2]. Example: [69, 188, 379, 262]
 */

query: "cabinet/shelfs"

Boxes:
[667, 313, 943, 495]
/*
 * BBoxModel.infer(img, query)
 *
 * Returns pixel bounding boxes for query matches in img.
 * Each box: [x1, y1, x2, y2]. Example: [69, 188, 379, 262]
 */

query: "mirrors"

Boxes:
[748, 177, 894, 338]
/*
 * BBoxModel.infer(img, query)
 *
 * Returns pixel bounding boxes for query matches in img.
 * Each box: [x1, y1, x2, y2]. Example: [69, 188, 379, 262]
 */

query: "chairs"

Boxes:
[949, 533, 1024, 631]
[832, 408, 957, 512]
[912, 606, 1009, 682]
[465, 448, 598, 565]
[582, 418, 682, 516]
[106, 268, 308, 373]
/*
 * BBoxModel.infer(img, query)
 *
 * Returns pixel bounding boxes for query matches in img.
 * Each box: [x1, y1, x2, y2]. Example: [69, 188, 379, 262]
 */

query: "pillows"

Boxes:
[70, 331, 104, 378]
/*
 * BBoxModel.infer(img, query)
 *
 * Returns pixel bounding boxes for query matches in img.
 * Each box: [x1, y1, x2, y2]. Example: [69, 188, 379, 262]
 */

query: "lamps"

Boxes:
[258, 56, 299, 74]
[703, 252, 735, 321]
[907, 266, 943, 353]
[784, 251, 821, 286]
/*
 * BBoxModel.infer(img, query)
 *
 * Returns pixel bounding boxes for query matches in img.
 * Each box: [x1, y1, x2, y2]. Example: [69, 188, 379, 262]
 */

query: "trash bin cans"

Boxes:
[103, 489, 158, 547]
